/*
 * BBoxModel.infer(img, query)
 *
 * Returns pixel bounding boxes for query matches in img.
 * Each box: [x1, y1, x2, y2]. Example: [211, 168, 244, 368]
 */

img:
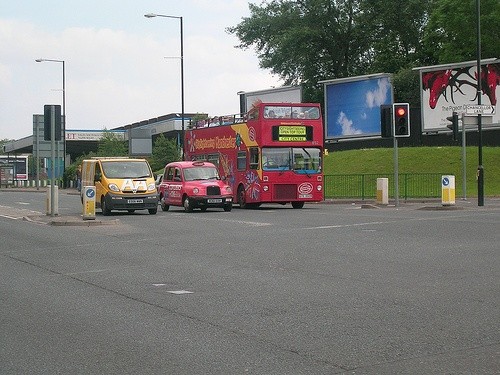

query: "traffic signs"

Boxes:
[463, 105, 497, 117]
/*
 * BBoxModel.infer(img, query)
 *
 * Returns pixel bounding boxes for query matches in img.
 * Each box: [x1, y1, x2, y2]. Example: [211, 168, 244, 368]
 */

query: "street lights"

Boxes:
[143, 12, 185, 159]
[36, 58, 66, 188]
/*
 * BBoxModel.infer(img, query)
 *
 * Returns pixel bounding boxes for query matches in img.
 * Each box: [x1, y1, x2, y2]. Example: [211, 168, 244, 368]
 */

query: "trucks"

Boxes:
[80, 156, 158, 215]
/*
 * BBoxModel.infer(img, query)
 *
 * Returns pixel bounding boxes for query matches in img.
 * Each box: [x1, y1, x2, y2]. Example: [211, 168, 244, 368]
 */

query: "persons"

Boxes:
[193, 111, 318, 128]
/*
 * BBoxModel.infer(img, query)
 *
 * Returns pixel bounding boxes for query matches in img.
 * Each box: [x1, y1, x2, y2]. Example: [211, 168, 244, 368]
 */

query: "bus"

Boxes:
[182, 102, 324, 208]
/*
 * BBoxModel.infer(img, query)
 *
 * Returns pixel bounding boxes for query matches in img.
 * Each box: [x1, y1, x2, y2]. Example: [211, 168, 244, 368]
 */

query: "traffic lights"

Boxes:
[445, 112, 459, 142]
[392, 102, 411, 137]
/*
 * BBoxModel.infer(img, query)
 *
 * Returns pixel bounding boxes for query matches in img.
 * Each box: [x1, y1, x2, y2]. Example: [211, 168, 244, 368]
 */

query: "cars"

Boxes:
[159, 161, 232, 213]
